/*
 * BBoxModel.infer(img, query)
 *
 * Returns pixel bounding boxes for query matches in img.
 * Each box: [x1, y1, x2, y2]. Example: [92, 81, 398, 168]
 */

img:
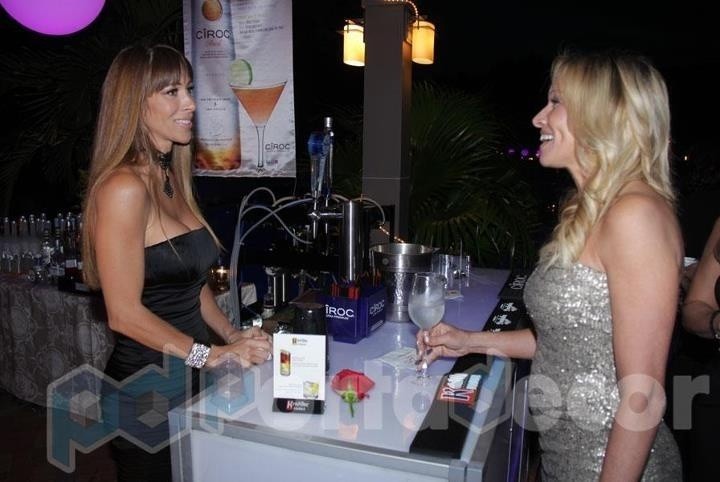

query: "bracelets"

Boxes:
[185, 337, 211, 369]
[710, 310, 719, 338]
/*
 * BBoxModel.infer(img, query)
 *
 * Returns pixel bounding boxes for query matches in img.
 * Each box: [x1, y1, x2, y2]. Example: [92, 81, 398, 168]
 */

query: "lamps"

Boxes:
[411, 14, 435, 65]
[341, 17, 365, 67]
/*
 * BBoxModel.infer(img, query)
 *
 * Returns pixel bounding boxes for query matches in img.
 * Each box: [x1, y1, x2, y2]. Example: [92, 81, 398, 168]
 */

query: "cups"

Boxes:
[437, 253, 454, 287]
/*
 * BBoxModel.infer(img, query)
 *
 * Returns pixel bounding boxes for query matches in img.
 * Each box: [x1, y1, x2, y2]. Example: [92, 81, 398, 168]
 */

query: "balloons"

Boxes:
[1, 1, 106, 38]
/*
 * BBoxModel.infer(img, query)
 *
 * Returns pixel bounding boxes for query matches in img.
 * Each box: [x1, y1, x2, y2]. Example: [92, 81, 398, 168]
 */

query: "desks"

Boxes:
[1, 276, 233, 424]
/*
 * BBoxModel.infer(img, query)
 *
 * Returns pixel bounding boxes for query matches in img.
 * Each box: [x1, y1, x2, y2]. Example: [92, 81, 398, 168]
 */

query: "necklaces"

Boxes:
[150, 147, 174, 198]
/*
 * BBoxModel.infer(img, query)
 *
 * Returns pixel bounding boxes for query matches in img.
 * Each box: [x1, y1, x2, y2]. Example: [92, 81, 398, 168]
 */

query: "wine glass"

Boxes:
[407, 271, 447, 388]
[231, 76, 289, 175]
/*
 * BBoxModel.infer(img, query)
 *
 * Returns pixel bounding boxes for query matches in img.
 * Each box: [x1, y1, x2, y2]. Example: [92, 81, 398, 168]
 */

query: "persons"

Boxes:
[414, 45, 687, 480]
[682, 218, 720, 339]
[82, 42, 276, 481]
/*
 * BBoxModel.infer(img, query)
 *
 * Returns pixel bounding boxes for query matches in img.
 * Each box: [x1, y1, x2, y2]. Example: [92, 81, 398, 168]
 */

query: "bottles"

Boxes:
[464, 254, 471, 277]
[190, 0, 241, 173]
[1, 212, 85, 279]
[294, 302, 329, 372]
